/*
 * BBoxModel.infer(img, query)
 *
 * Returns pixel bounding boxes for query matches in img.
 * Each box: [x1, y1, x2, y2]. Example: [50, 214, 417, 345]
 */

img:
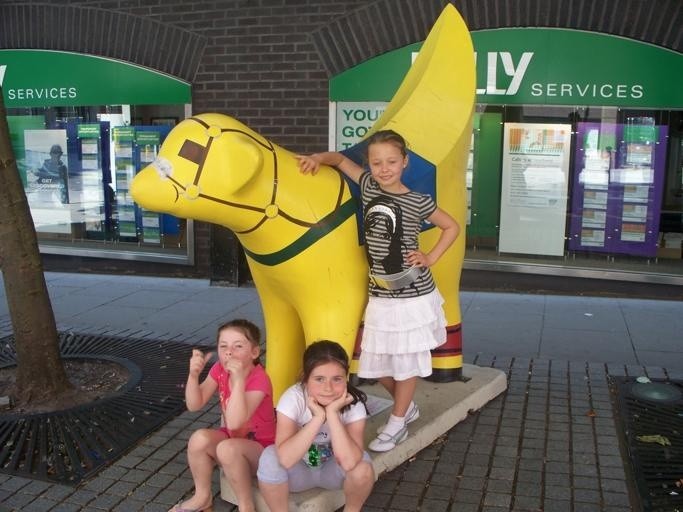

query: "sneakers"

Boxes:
[369, 400, 419, 452]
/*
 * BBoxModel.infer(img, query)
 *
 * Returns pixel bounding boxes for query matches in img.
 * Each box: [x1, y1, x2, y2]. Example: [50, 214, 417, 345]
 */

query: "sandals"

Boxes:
[167, 500, 213, 512]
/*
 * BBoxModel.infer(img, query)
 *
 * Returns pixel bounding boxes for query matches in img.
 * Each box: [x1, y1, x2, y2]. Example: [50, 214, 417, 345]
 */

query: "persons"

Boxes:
[164, 319, 277, 512]
[256, 340, 375, 512]
[294, 129, 460, 452]
[36, 143, 68, 205]
